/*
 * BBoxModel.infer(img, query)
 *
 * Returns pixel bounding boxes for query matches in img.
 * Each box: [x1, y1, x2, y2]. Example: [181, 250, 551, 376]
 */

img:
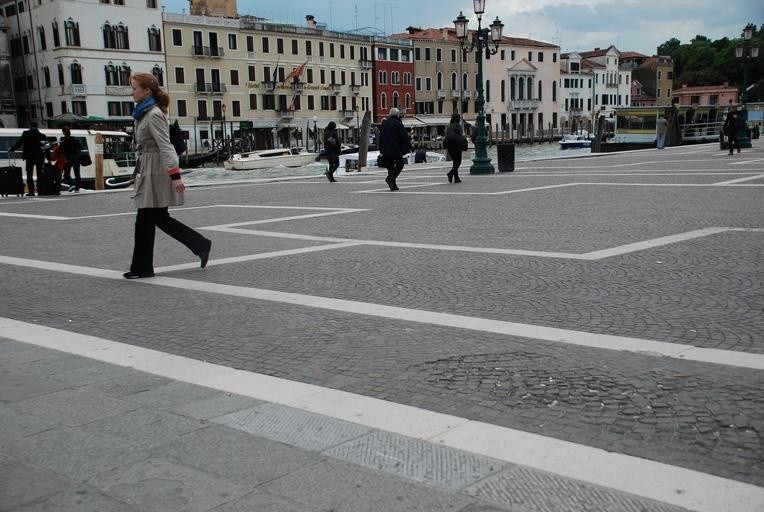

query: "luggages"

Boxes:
[0, 151, 24, 197]
[39, 160, 62, 195]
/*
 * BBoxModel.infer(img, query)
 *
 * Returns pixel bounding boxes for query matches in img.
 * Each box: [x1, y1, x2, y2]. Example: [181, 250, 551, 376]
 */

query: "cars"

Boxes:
[572, 129, 594, 138]
[318, 142, 360, 155]
[432, 134, 443, 141]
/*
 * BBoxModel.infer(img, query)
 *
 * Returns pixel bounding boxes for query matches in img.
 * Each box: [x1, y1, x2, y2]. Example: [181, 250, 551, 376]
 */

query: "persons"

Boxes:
[378, 108, 414, 192]
[323, 121, 341, 183]
[56, 124, 85, 194]
[655, 114, 669, 150]
[721, 103, 744, 156]
[377, 111, 405, 169]
[441, 111, 469, 184]
[6, 119, 55, 198]
[120, 72, 212, 279]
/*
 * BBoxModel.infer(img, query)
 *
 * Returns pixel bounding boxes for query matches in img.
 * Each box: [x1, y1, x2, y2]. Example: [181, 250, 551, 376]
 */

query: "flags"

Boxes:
[271, 65, 278, 92]
[284, 62, 306, 84]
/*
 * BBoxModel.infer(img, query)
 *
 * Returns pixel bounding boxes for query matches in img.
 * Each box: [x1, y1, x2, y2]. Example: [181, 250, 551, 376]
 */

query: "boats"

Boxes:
[558, 133, 592, 149]
[589, 103, 742, 153]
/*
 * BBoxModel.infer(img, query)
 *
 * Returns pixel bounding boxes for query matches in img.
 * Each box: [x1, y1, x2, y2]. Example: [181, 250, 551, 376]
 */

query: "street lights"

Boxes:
[453, 0, 506, 175]
[589, 69, 595, 134]
[221, 101, 228, 144]
[355, 105, 360, 146]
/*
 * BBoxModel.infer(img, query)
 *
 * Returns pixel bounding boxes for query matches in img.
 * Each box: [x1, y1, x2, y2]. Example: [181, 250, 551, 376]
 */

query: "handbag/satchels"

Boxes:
[168, 119, 186, 154]
[79, 152, 92, 166]
[326, 137, 341, 154]
[443, 134, 468, 151]
[377, 155, 386, 167]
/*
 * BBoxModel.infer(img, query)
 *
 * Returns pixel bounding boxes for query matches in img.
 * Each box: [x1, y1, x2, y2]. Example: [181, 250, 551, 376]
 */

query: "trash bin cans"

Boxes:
[720, 131, 729, 148]
[497, 144, 515, 171]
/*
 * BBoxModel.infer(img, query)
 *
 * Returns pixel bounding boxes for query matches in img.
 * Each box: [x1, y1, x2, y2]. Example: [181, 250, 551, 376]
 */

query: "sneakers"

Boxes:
[325, 172, 336, 182]
[386, 177, 399, 190]
[447, 173, 461, 183]
[26, 193, 35, 196]
[198, 240, 211, 267]
[123, 270, 155, 279]
[68, 184, 79, 193]
